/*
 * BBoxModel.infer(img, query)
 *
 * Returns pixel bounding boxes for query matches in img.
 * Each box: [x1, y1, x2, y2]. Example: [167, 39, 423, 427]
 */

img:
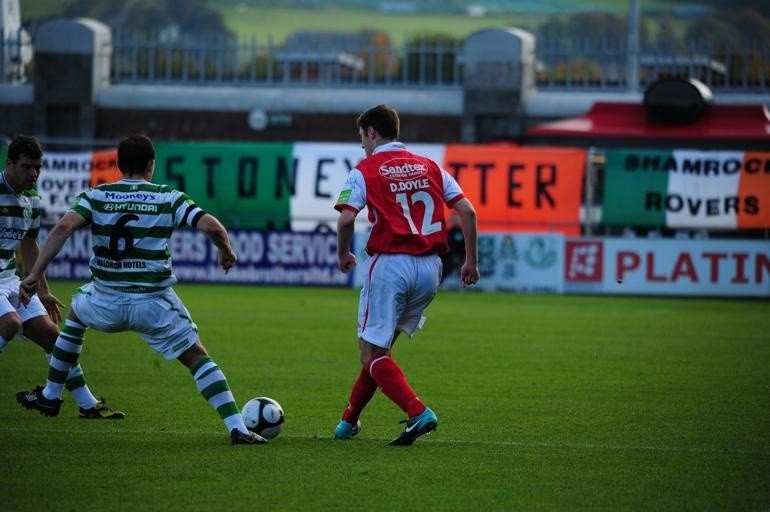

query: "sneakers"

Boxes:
[15, 385, 65, 417]
[334, 419, 362, 439]
[79, 403, 126, 420]
[230, 428, 269, 444]
[387, 407, 438, 446]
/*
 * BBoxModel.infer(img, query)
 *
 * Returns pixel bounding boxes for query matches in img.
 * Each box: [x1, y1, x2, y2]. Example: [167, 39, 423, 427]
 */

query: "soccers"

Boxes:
[241, 397, 285, 441]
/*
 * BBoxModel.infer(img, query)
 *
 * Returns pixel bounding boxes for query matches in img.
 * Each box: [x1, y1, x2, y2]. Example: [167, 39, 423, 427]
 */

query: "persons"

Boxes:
[0, 135, 126, 419]
[334, 104, 480, 445]
[15, 135, 269, 444]
